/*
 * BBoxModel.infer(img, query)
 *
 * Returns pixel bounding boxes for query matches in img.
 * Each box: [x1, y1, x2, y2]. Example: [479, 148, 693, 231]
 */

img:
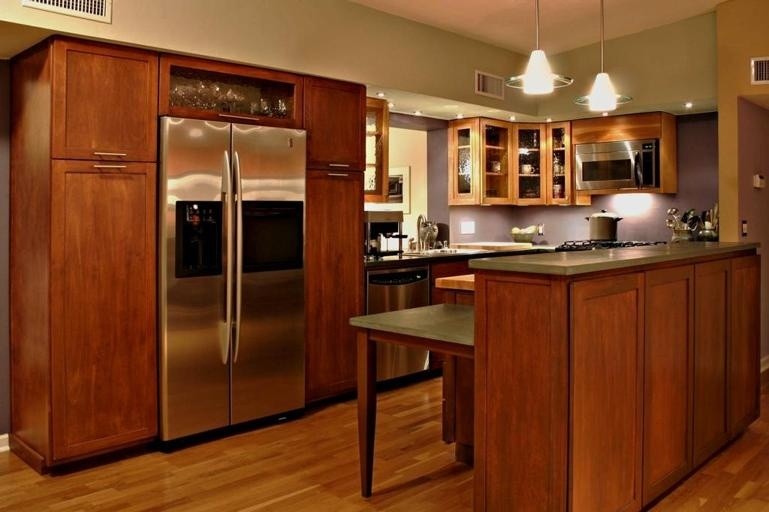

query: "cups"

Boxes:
[554, 184, 563, 198]
[521, 164, 535, 174]
[671, 229, 694, 244]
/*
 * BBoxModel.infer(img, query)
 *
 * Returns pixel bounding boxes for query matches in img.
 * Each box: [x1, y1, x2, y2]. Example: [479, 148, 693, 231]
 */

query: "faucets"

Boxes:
[416, 215, 433, 251]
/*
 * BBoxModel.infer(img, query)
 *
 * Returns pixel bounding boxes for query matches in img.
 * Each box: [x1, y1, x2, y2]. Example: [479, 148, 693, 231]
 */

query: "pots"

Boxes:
[584, 208, 624, 241]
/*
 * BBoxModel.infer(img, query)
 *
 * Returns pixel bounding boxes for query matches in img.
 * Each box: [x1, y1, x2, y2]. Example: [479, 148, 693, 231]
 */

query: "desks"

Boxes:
[348, 274, 476, 498]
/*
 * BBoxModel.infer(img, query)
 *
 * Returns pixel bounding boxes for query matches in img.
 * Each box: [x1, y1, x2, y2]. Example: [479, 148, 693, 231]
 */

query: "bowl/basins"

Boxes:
[510, 232, 534, 243]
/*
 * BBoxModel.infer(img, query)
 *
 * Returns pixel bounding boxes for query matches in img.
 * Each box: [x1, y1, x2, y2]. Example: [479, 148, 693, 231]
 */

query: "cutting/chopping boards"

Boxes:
[449, 241, 533, 252]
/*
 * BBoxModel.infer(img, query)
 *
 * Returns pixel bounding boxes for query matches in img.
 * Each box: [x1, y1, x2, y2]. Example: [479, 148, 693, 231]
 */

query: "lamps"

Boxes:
[504, 0, 577, 97]
[572, 0, 634, 113]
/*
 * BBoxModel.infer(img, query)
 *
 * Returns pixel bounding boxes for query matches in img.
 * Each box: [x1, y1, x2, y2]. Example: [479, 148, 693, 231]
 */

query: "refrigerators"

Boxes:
[158, 115, 308, 453]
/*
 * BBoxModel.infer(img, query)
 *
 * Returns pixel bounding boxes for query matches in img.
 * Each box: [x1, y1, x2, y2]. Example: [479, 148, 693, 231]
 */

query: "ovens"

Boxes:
[364, 267, 434, 382]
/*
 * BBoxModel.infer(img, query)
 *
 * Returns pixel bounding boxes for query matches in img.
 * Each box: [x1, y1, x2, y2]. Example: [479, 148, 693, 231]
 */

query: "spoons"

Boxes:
[686, 207, 696, 219]
[665, 219, 675, 229]
[666, 207, 679, 219]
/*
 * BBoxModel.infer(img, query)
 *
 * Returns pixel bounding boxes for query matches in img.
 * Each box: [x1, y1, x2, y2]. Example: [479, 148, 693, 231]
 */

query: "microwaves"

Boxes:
[575, 138, 660, 191]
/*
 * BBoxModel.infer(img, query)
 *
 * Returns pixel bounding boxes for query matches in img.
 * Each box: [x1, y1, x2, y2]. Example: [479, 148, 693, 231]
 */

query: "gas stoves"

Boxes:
[555, 240, 667, 252]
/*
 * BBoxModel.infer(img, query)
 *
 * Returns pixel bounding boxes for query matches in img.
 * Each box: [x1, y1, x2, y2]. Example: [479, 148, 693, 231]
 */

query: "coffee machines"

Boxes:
[363, 211, 407, 260]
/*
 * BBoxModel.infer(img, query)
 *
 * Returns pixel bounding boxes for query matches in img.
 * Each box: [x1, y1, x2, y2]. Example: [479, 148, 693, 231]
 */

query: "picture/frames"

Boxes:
[365, 165, 412, 215]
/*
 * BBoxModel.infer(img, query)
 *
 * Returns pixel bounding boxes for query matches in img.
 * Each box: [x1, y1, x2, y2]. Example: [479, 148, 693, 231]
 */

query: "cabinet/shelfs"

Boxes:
[446, 116, 511, 207]
[469, 248, 763, 512]
[9, 34, 160, 477]
[571, 110, 679, 207]
[441, 289, 473, 467]
[304, 76, 365, 404]
[512, 120, 571, 208]
[365, 96, 390, 205]
